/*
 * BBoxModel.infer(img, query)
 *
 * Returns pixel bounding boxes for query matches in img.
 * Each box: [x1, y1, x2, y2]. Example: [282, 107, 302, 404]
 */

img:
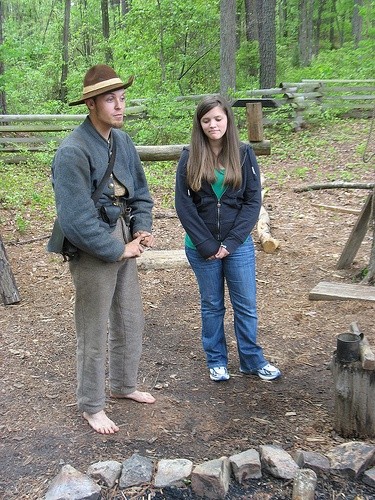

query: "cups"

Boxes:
[336, 333, 364, 363]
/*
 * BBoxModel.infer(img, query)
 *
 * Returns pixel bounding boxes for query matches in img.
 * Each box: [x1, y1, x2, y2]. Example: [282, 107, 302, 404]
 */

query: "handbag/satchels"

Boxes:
[47, 217, 79, 260]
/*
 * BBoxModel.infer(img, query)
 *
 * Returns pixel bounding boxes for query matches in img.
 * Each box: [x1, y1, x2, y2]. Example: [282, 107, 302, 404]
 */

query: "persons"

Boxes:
[51, 65, 156, 435]
[175, 95, 280, 382]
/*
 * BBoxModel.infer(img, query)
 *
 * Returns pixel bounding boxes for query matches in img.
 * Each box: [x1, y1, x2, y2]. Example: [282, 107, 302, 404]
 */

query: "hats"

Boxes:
[68, 63, 135, 107]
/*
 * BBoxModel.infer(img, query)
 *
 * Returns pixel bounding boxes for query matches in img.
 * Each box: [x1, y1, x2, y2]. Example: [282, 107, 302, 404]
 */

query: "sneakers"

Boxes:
[239, 362, 282, 381]
[209, 366, 230, 382]
